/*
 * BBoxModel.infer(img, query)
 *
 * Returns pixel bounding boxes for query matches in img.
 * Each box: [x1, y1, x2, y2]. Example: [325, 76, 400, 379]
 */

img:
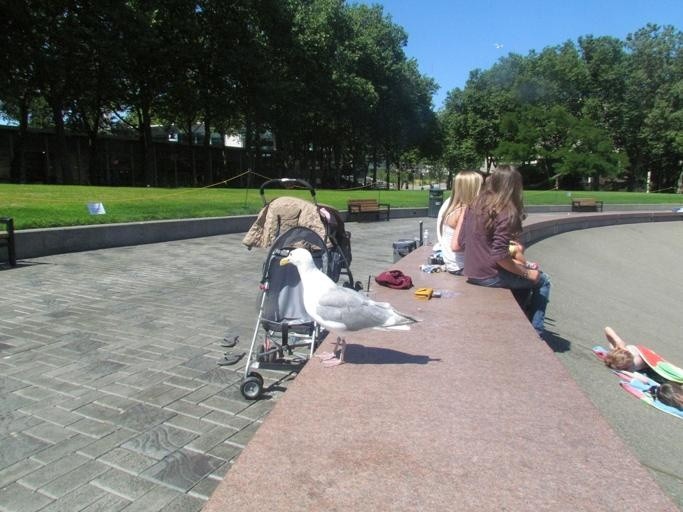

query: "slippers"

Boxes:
[217, 349, 245, 365]
[221, 334, 239, 347]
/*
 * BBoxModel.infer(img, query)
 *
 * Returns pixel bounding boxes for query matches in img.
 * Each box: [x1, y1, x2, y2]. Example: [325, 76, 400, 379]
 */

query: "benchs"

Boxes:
[572, 199, 603, 211]
[0, 218, 16, 266]
[348, 199, 390, 223]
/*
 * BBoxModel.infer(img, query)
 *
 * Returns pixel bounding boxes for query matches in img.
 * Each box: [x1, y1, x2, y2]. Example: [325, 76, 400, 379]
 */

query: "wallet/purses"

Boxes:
[414, 287, 432, 301]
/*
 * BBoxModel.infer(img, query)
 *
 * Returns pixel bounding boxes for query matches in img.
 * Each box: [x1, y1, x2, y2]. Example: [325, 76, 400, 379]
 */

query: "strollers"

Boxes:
[239, 179, 364, 400]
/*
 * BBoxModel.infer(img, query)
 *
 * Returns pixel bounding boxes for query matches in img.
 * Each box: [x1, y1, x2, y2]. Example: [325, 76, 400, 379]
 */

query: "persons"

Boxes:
[656, 381, 683, 411]
[508, 240, 538, 270]
[436, 168, 484, 277]
[458, 164, 551, 337]
[603, 326, 648, 372]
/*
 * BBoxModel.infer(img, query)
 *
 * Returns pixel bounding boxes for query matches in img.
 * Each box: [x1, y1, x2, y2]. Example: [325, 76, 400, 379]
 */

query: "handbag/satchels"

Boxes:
[375, 270, 412, 290]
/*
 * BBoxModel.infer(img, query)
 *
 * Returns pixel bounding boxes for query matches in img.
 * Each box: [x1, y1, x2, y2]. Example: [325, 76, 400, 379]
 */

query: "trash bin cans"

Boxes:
[428, 189, 445, 217]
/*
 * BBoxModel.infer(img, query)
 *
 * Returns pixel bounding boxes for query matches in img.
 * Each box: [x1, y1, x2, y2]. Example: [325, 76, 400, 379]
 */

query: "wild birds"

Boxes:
[280, 247, 423, 367]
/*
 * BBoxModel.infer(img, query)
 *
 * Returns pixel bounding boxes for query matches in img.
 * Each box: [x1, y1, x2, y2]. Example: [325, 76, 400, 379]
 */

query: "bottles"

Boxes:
[423, 229, 428, 246]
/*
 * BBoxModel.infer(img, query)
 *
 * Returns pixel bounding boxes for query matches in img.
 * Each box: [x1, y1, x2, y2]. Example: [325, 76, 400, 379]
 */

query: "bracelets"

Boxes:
[520, 270, 528, 280]
